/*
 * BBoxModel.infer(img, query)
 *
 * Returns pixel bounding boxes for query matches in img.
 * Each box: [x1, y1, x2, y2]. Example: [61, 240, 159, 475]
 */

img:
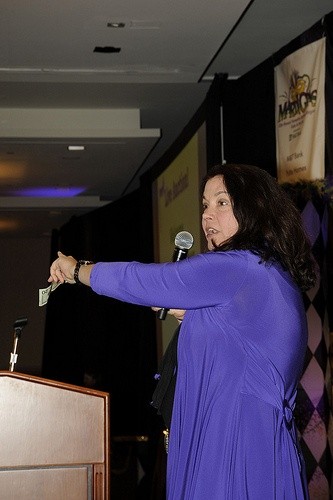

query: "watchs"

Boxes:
[74, 260, 93, 283]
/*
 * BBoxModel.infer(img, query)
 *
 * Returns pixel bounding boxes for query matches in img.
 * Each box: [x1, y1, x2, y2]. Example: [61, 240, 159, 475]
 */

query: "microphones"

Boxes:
[159, 232, 193, 320]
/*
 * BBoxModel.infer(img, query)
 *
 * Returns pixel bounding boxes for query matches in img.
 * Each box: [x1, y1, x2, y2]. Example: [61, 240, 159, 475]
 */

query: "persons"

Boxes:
[48, 162, 318, 500]
[82, 370, 106, 391]
